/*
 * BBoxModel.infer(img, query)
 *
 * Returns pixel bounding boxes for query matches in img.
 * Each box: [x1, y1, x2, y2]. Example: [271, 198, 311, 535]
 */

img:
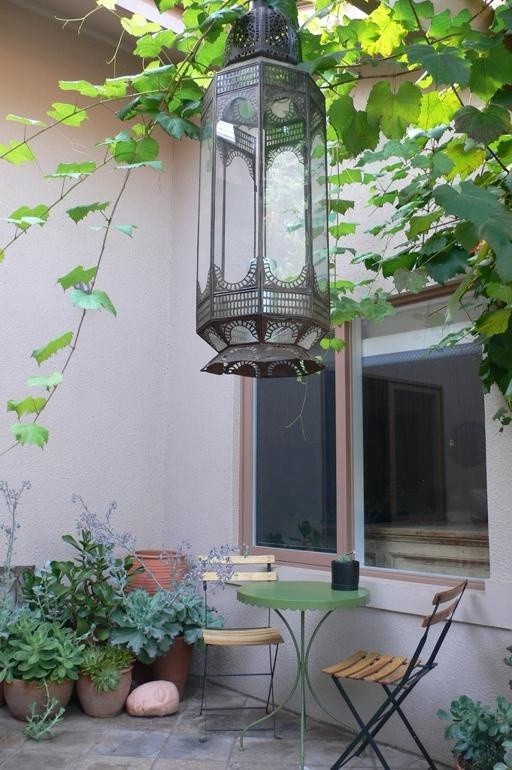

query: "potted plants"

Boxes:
[77, 645, 137, 718]
[0, 614, 87, 722]
[331, 553, 359, 591]
[112, 587, 226, 703]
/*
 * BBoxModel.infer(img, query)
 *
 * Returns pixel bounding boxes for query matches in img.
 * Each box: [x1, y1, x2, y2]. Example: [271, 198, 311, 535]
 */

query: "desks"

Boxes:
[237, 580, 371, 770]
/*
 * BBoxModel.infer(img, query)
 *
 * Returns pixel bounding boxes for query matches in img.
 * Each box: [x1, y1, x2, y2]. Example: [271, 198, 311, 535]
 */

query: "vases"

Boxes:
[123, 551, 190, 598]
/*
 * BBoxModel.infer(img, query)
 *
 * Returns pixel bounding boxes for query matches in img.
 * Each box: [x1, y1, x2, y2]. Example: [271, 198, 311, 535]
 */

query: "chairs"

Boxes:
[320, 579, 468, 770]
[197, 555, 284, 743]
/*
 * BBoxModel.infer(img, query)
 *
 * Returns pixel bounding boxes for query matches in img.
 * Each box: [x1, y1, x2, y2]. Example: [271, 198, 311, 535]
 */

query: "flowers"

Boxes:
[73, 494, 250, 605]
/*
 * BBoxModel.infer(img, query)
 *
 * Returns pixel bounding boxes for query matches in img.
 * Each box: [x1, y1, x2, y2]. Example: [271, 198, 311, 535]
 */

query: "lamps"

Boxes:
[196, 0, 330, 377]
